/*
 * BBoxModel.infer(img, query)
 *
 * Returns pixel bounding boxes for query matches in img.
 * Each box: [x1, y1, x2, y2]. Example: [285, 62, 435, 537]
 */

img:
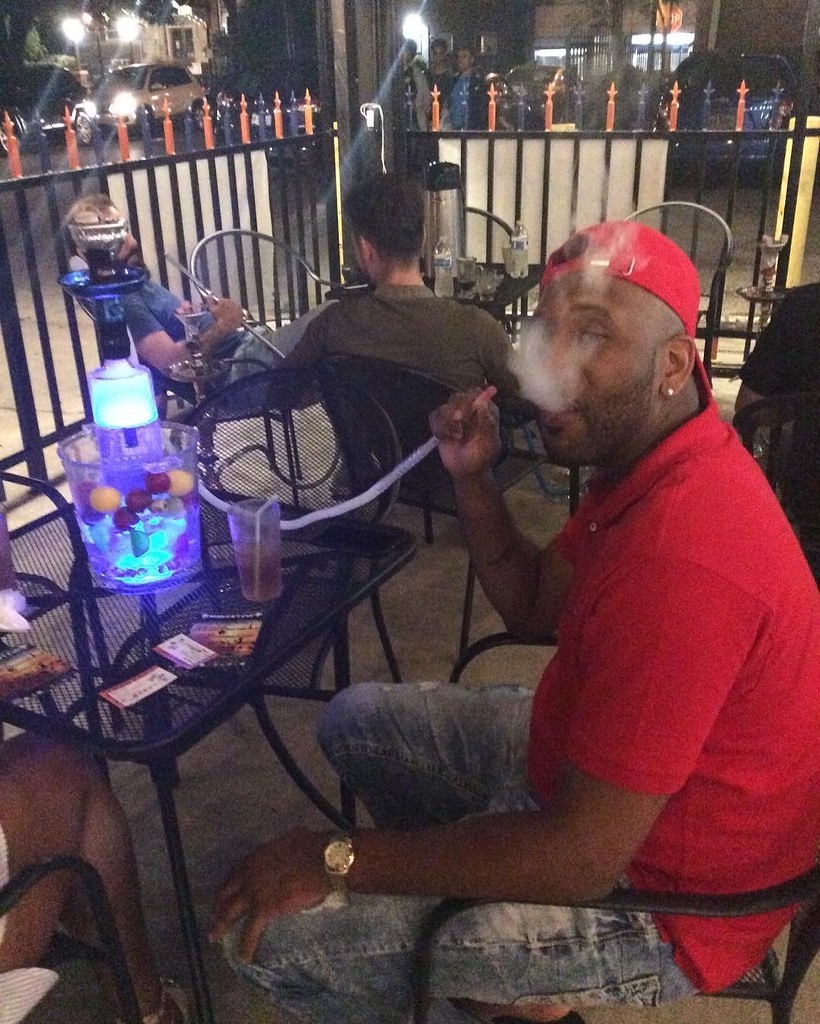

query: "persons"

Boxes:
[59, 192, 354, 501]
[732, 284, 820, 593]
[0, 731, 188, 1024]
[402, 38, 489, 130]
[207, 221, 820, 1024]
[270, 173, 538, 495]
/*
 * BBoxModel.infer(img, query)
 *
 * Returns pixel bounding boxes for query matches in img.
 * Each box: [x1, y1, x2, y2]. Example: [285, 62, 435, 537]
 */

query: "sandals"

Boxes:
[142, 975, 188, 1024]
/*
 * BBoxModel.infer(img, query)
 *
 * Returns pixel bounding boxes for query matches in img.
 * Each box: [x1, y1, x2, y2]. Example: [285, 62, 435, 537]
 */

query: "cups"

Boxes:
[473, 269, 496, 301]
[456, 256, 477, 283]
[228, 498, 282, 602]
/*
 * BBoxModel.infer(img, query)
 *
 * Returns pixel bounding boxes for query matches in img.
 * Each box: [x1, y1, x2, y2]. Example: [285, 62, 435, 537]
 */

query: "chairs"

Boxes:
[0, 201, 820, 1024]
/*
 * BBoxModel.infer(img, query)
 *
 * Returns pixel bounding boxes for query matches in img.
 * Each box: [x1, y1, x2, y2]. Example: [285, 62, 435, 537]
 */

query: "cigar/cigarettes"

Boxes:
[464, 385, 498, 423]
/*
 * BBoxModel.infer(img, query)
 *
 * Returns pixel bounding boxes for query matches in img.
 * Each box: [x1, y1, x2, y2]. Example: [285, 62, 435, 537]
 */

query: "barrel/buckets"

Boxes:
[56, 423, 202, 594]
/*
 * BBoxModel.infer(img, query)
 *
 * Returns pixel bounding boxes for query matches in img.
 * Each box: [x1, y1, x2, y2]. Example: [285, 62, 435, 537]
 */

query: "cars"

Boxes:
[505, 64, 586, 128]
[664, 46, 800, 184]
[87, 64, 208, 139]
[196, 61, 321, 138]
[0, 64, 89, 155]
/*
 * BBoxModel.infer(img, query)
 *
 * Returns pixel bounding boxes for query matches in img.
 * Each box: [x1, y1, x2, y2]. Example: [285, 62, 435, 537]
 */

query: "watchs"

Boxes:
[316, 828, 356, 897]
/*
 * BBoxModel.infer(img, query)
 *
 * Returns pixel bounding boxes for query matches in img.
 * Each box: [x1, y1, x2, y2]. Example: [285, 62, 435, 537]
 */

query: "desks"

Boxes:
[0, 488, 416, 1024]
[326, 261, 547, 353]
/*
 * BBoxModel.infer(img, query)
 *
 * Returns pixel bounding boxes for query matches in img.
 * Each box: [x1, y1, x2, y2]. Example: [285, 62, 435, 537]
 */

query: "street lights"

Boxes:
[81, 12, 108, 77]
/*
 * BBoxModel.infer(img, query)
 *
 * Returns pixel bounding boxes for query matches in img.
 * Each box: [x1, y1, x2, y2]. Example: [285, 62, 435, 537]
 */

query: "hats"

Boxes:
[539, 219, 713, 398]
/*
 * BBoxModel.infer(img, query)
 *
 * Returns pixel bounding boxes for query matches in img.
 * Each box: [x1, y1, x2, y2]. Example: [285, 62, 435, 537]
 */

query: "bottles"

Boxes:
[511, 220, 529, 279]
[502, 229, 513, 275]
[433, 235, 454, 298]
